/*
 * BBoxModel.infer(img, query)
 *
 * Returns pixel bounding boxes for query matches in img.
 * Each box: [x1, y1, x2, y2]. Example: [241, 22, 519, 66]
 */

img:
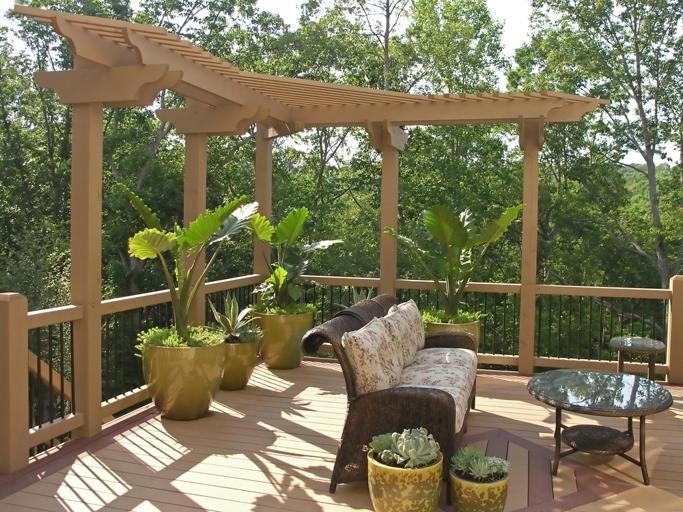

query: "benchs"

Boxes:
[302, 292, 477, 506]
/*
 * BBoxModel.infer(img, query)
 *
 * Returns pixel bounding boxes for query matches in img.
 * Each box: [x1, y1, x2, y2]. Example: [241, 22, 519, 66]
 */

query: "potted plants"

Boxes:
[114, 181, 259, 420]
[245, 206, 344, 370]
[366, 433, 513, 510]
[207, 291, 264, 390]
[383, 201, 528, 338]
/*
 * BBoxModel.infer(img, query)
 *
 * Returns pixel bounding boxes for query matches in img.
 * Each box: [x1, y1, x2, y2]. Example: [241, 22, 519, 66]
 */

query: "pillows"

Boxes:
[341, 297, 426, 398]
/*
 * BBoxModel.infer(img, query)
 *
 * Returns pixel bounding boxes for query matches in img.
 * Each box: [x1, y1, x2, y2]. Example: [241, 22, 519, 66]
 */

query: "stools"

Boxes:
[611, 336, 665, 384]
